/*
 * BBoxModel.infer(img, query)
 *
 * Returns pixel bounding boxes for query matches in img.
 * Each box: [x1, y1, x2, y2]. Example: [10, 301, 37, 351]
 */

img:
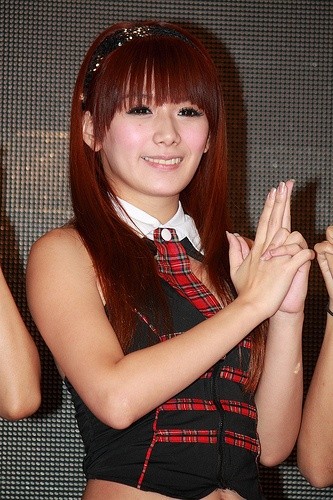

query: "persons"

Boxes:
[295, 222, 333, 489]
[22, 15, 316, 500]
[0, 258, 43, 421]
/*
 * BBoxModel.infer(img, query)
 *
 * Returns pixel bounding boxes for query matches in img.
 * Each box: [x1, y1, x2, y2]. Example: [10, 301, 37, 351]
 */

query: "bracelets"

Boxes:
[324, 302, 333, 318]
